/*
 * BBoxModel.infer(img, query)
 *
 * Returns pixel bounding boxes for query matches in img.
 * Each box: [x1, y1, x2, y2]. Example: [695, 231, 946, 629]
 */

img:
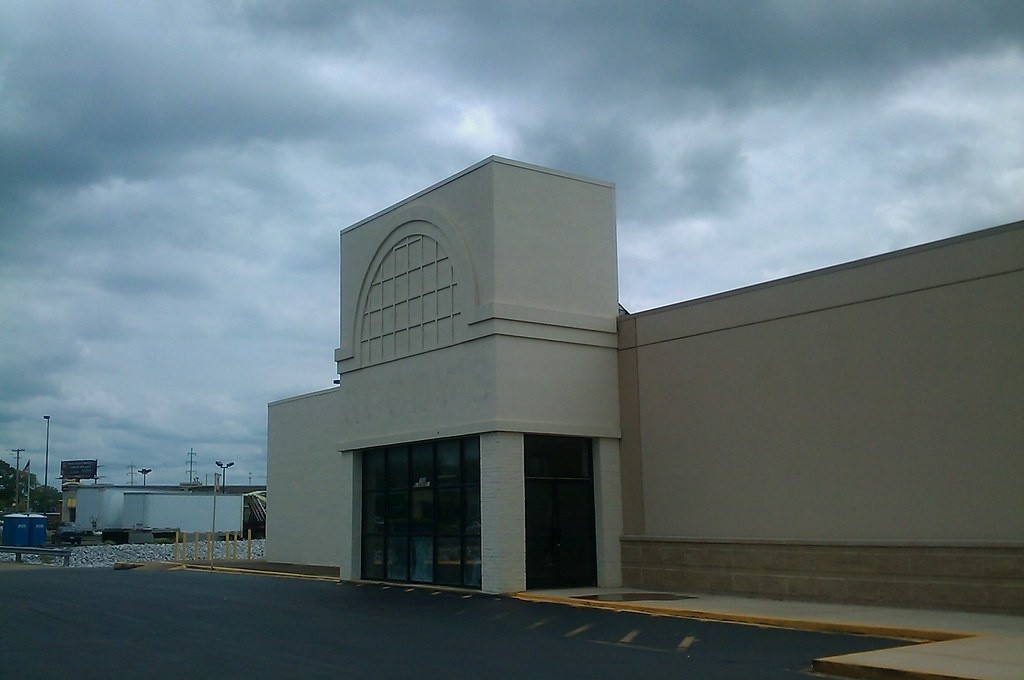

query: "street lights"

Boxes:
[43, 415, 51, 516]
[138, 468, 152, 486]
[216, 461, 234, 494]
[12, 449, 25, 513]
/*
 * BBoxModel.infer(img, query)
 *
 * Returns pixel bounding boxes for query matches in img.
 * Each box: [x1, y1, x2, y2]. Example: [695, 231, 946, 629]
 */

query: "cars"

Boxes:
[53, 522, 82, 545]
